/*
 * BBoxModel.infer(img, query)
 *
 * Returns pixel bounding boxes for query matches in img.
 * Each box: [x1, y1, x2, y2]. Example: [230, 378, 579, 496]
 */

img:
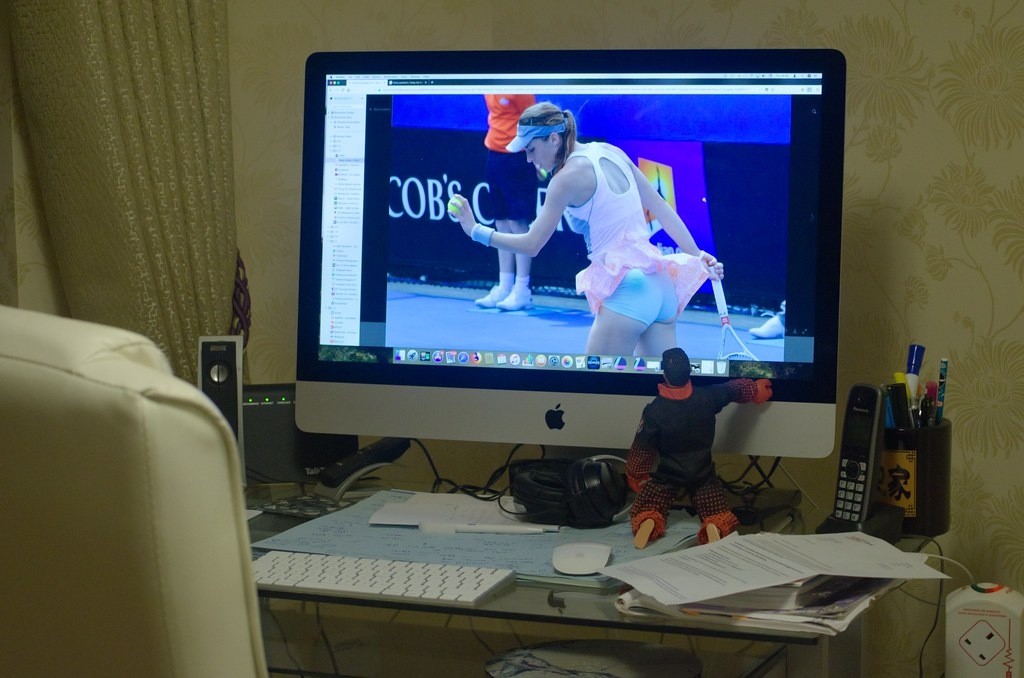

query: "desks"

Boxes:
[245, 481, 937, 678]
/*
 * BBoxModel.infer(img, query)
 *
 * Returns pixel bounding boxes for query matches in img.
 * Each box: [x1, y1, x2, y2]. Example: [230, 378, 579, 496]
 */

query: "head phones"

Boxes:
[513, 454, 638, 528]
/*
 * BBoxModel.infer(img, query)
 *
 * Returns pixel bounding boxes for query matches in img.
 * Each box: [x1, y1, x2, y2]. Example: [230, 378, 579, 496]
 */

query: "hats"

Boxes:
[506, 123, 566, 152]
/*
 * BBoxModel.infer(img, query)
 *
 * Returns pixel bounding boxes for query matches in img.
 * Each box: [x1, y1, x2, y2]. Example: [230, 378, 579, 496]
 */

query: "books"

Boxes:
[616, 552, 930, 637]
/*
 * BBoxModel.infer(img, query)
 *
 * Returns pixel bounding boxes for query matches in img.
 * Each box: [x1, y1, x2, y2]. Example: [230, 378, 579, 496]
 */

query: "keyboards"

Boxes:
[250, 550, 515, 610]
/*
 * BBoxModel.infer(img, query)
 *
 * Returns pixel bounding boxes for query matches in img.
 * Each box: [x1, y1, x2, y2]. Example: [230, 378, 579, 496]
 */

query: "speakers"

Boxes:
[196, 334, 245, 488]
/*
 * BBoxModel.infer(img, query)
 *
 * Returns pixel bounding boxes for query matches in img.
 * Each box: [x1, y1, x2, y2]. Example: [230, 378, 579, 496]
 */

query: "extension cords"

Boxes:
[945, 580, 1024, 678]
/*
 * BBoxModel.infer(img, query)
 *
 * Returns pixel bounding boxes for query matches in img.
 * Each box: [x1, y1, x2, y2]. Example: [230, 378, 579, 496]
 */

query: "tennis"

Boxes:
[448, 197, 464, 215]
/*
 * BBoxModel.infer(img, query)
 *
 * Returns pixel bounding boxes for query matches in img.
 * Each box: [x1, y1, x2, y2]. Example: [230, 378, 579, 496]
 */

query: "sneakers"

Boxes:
[475, 285, 513, 307]
[496, 290, 534, 311]
[749, 315, 783, 338]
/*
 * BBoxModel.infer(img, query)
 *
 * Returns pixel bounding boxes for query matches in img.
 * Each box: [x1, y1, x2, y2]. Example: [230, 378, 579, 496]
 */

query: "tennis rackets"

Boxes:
[709, 265, 758, 361]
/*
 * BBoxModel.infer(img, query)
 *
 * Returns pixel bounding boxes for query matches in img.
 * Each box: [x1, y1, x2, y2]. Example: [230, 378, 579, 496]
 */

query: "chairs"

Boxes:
[0, 304, 270, 678]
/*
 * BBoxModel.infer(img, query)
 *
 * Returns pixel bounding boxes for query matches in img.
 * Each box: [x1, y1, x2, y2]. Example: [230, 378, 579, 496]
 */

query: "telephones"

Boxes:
[812, 382, 904, 544]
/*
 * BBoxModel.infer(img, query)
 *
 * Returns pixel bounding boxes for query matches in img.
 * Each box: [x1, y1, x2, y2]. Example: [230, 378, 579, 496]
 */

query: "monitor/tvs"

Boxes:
[292, 47, 847, 532]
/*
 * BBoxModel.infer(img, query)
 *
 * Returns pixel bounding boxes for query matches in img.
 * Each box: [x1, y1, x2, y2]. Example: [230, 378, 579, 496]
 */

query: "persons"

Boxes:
[699, 250, 704, 261]
[750, 296, 786, 341]
[474, 94, 537, 310]
[447, 102, 725, 356]
[626, 348, 772, 549]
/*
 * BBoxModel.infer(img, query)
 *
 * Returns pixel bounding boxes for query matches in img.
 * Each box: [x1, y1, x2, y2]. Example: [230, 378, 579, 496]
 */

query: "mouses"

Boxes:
[552, 540, 612, 576]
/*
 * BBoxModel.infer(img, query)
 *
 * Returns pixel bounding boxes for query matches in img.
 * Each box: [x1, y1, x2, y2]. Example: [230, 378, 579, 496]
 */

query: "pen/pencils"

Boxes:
[880, 344, 949, 428]
